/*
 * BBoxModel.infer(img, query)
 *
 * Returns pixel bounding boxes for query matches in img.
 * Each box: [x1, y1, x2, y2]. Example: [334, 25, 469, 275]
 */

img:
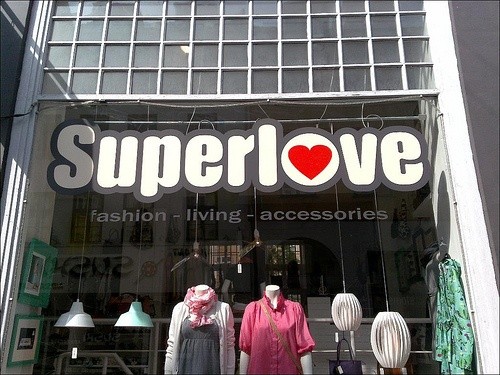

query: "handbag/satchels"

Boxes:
[329, 339, 363, 375]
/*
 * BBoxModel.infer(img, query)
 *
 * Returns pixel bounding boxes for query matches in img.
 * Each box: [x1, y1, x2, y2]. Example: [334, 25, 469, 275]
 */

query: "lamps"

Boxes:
[170, 192, 215, 274]
[114, 203, 154, 328]
[238, 186, 275, 261]
[370, 189, 411, 368]
[54, 189, 95, 327]
[331, 184, 362, 331]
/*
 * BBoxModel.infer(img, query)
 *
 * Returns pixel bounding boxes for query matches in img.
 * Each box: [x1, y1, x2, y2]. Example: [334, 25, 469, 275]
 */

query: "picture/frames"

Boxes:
[394, 227, 436, 294]
[7, 313, 44, 366]
[17, 237, 60, 309]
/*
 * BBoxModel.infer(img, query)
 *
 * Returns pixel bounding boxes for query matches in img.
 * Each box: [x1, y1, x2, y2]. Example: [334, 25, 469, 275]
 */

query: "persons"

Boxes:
[221, 248, 266, 325]
[239, 285, 316, 375]
[163, 285, 235, 375]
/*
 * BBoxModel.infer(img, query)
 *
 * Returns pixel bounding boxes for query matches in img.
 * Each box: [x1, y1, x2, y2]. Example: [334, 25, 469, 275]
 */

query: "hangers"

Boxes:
[439, 242, 450, 263]
